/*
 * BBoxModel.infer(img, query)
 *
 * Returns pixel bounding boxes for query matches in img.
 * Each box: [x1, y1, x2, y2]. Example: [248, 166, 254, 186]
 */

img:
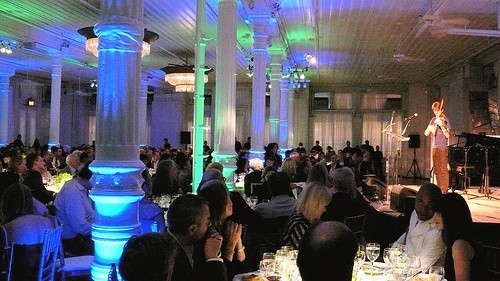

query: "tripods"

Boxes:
[405, 148, 422, 181]
[459, 148, 500, 200]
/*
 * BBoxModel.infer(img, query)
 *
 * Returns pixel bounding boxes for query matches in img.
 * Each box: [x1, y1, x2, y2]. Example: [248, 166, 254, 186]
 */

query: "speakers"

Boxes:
[408, 134, 420, 148]
[181, 132, 191, 144]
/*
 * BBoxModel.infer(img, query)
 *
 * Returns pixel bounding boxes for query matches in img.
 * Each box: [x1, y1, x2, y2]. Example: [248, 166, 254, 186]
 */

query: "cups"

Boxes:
[43, 177, 55, 186]
[260, 246, 302, 281]
[155, 194, 183, 206]
[383, 243, 444, 281]
[357, 245, 364, 250]
[352, 272, 365, 281]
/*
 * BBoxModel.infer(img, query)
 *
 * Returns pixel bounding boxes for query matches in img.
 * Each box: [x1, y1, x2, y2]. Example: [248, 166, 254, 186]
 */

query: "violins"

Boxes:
[435, 116, 448, 129]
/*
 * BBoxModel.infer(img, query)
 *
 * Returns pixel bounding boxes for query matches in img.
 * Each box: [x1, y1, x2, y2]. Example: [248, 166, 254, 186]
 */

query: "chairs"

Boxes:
[1, 214, 93, 281]
[244, 48, 313, 97]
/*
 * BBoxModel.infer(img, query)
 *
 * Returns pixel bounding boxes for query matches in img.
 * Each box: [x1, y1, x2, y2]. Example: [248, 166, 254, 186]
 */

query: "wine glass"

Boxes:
[366, 242, 380, 266]
[249, 196, 258, 208]
[353, 251, 365, 272]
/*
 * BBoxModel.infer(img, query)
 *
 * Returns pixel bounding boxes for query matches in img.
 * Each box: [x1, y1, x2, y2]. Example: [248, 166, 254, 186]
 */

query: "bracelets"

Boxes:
[236, 246, 245, 252]
[225, 248, 235, 254]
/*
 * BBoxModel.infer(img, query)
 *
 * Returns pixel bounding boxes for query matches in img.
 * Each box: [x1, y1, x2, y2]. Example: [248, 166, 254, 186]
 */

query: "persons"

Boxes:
[0, 135, 95, 257]
[433, 193, 484, 281]
[118, 137, 383, 281]
[296, 221, 358, 281]
[392, 183, 446, 274]
[424, 102, 450, 193]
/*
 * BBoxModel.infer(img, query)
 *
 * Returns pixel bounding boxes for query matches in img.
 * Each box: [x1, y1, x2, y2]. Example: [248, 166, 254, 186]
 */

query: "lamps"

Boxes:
[160, 59, 212, 93]
[77, 26, 159, 58]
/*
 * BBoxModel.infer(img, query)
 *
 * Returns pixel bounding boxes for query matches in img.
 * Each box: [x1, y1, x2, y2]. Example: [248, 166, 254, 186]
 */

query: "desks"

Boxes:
[233, 261, 446, 281]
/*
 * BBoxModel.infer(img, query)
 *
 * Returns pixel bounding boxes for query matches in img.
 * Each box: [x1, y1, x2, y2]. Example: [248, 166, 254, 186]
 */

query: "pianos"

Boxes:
[455, 132, 500, 191]
